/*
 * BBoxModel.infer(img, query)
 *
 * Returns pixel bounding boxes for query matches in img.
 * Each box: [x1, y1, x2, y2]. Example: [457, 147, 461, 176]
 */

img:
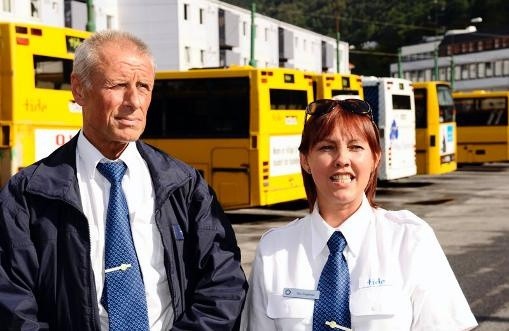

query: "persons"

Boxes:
[0, 31, 249, 331]
[240, 100, 478, 331]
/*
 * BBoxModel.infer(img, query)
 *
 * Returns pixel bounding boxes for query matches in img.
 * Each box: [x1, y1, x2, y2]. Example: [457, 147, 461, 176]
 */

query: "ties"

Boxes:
[96, 162, 150, 331]
[312, 231, 351, 331]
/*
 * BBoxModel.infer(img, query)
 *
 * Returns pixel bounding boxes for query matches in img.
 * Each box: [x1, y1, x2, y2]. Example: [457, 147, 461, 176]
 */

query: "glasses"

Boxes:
[304, 99, 373, 125]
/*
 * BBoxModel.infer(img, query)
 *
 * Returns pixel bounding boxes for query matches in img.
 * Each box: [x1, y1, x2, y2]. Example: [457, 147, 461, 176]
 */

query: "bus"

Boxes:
[138, 66, 315, 213]
[0, 22, 100, 193]
[311, 72, 365, 104]
[413, 81, 457, 177]
[361, 76, 417, 182]
[452, 92, 509, 166]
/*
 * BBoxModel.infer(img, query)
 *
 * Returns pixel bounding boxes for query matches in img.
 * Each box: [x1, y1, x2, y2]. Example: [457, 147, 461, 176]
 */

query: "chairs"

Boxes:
[298, 105, 302, 109]
[35, 80, 55, 90]
[494, 109, 504, 125]
[271, 104, 276, 108]
[59, 83, 73, 90]
[278, 104, 286, 109]
[486, 111, 495, 126]
[288, 104, 296, 110]
